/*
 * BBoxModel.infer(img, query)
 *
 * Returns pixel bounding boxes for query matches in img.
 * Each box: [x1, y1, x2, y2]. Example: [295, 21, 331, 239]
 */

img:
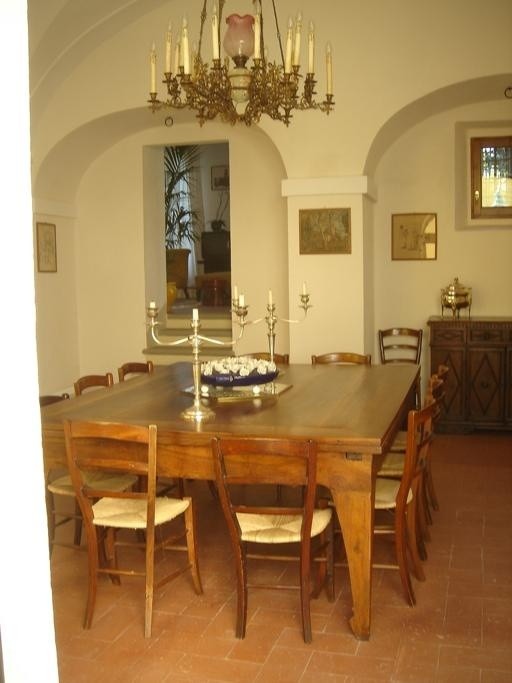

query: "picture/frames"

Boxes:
[392, 214, 438, 260]
[298, 208, 352, 254]
[37, 223, 57, 271]
[470, 137, 512, 219]
[211, 165, 229, 190]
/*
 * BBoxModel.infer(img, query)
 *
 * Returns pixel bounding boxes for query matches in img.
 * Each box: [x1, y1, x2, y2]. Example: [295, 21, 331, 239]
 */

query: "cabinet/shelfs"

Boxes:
[427, 316, 512, 434]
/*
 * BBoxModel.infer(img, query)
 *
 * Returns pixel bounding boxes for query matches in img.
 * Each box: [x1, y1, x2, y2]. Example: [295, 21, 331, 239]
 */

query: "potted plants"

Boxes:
[165, 145, 201, 289]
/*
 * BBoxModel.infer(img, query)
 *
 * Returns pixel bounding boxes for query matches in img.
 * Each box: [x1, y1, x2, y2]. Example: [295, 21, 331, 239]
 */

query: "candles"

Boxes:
[193, 308, 199, 320]
[150, 301, 155, 308]
[269, 289, 273, 310]
[235, 286, 238, 298]
[303, 282, 307, 296]
[239, 295, 244, 307]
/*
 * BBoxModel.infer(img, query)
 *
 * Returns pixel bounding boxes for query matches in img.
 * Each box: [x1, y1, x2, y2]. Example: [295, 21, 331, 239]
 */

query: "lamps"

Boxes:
[147, 0, 335, 128]
